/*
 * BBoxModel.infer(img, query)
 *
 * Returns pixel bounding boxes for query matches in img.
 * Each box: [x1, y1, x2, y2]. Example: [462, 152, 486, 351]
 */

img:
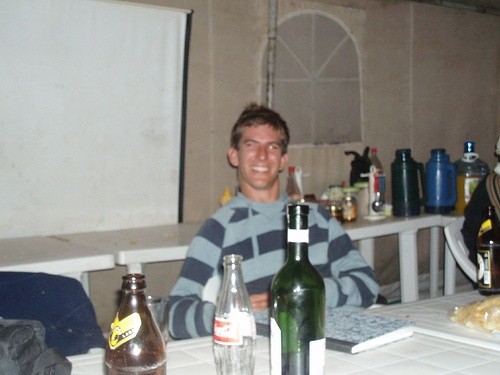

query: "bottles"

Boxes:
[102, 271, 168, 375]
[326, 182, 369, 223]
[284, 166, 302, 202]
[476, 205, 500, 296]
[268, 204, 327, 375]
[212, 254, 256, 375]
[369, 148, 385, 205]
[453, 141, 489, 215]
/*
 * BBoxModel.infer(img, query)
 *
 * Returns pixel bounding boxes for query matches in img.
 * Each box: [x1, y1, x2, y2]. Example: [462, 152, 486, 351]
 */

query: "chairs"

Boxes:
[0, 271, 108, 357]
[445, 215, 478, 282]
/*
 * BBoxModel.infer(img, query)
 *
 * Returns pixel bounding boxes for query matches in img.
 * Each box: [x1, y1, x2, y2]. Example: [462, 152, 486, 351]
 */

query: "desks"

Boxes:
[0, 205, 500, 375]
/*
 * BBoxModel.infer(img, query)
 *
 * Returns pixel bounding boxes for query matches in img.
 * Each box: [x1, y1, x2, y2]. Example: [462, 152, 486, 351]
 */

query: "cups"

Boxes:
[147, 296, 168, 345]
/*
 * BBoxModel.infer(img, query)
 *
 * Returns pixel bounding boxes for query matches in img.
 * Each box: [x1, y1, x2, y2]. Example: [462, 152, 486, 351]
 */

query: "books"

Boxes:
[253, 308, 415, 353]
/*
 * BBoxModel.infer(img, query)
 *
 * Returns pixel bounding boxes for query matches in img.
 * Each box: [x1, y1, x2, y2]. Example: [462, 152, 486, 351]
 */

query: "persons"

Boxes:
[156, 103, 379, 338]
[461, 138, 500, 266]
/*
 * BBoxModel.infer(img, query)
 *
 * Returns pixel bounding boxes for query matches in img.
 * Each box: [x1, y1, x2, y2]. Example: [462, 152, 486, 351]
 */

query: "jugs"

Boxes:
[391, 149, 427, 217]
[424, 148, 457, 215]
